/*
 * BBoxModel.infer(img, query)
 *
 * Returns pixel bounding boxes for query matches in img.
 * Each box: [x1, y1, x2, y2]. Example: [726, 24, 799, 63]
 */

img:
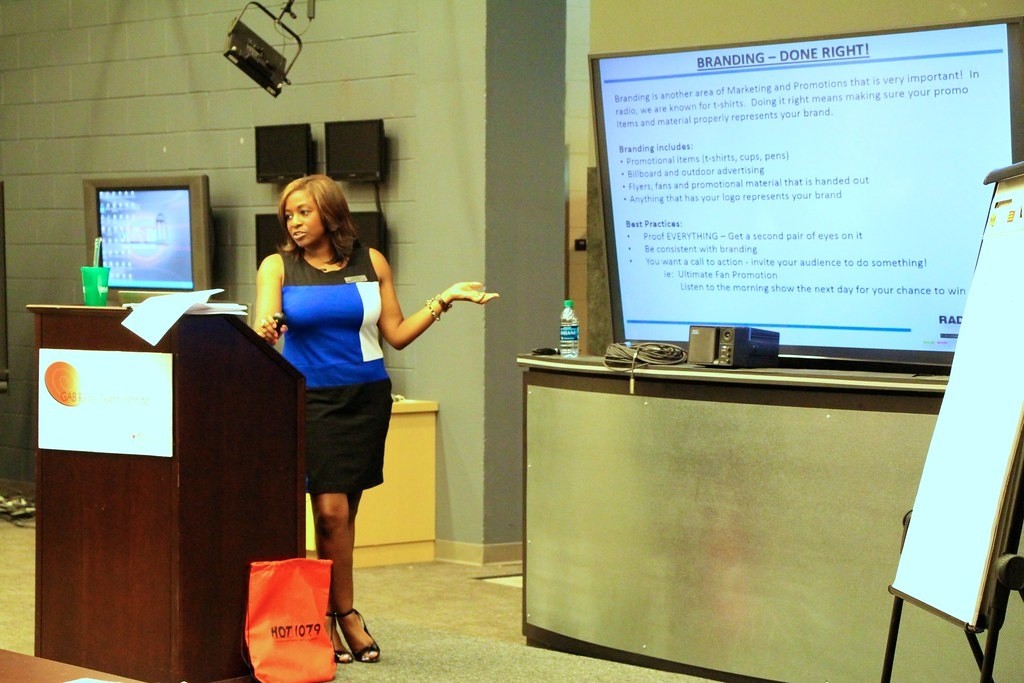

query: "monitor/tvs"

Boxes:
[323, 118, 388, 187]
[77, 173, 227, 311]
[251, 122, 321, 190]
[255, 210, 383, 284]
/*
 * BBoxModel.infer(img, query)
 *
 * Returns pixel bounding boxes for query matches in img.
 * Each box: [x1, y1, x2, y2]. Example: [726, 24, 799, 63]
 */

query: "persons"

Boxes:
[252, 176, 499, 663]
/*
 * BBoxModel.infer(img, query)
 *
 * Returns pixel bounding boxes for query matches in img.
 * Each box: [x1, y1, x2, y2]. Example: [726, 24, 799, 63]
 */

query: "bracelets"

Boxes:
[435, 295, 452, 312]
[424, 298, 441, 321]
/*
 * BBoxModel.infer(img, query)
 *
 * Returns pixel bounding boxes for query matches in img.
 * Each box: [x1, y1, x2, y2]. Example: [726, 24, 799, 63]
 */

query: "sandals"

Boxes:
[336, 609, 380, 662]
[325, 609, 353, 663]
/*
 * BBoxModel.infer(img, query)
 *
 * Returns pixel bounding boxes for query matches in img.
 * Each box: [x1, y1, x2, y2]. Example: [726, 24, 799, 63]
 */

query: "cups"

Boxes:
[80, 266, 111, 307]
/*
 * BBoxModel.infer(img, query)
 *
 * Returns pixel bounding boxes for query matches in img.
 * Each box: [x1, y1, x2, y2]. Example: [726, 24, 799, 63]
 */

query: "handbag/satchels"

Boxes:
[244, 558, 336, 683]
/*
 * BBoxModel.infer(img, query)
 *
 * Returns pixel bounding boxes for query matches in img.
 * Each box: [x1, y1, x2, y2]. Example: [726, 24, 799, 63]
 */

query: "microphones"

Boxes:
[273, 312, 286, 337]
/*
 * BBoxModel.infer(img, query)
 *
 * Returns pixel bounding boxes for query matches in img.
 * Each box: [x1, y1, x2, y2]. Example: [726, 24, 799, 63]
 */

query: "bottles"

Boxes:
[560, 300, 580, 358]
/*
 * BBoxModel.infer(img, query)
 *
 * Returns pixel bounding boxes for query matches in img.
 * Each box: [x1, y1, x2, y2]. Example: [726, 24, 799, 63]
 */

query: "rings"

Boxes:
[260, 320, 266, 326]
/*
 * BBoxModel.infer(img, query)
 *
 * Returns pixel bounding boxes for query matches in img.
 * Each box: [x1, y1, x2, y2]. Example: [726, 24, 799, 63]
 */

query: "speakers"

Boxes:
[687, 325, 781, 369]
